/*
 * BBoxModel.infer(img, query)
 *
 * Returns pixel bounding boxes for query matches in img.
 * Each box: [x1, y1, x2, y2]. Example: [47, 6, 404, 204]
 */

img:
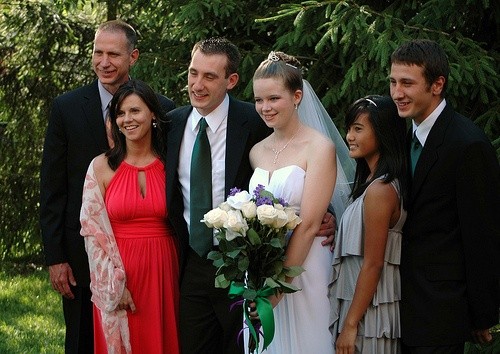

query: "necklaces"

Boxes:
[272, 124, 302, 162]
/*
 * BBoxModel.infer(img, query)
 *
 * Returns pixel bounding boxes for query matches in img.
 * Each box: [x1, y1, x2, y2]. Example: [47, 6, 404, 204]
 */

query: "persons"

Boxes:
[40, 21, 176, 354]
[152, 38, 336, 354]
[79, 80, 181, 354]
[328, 95, 408, 354]
[243, 50, 338, 354]
[389, 40, 500, 354]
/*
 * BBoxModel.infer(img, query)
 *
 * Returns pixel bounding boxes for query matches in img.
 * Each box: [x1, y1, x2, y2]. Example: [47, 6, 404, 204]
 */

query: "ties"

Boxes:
[105, 102, 115, 149]
[189, 118, 213, 257]
[411, 130, 422, 176]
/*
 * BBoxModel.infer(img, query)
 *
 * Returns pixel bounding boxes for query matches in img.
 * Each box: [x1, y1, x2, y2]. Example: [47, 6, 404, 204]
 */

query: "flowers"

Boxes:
[200, 184, 306, 354]
